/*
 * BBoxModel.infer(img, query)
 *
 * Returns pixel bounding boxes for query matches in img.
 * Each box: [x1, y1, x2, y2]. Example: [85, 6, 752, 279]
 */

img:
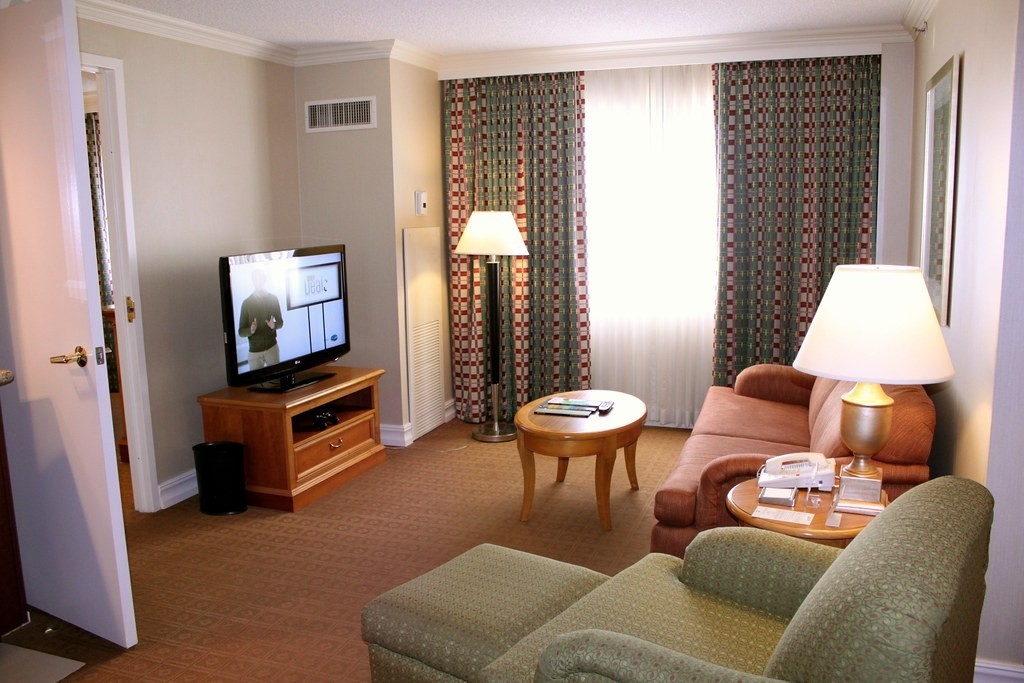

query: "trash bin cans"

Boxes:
[192, 440, 250, 515]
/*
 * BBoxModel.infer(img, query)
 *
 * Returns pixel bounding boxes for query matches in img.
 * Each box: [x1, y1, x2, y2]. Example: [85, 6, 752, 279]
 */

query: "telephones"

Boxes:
[758, 451, 837, 492]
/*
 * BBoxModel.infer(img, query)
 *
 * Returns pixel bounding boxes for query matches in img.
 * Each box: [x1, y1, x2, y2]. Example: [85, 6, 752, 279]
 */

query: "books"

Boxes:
[533, 397, 603, 418]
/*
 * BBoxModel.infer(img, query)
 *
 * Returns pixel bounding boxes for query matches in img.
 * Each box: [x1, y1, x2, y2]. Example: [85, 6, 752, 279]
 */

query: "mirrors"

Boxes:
[919, 51, 960, 328]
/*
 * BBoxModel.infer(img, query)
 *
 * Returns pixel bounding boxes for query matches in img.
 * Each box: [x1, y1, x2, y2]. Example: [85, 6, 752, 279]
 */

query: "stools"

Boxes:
[360, 542, 612, 683]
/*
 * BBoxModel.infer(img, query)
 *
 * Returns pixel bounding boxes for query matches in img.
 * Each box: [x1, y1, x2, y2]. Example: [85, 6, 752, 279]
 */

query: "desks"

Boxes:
[726, 473, 890, 549]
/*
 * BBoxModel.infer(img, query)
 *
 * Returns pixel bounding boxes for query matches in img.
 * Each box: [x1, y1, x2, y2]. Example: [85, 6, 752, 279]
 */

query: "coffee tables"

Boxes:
[513, 389, 647, 533]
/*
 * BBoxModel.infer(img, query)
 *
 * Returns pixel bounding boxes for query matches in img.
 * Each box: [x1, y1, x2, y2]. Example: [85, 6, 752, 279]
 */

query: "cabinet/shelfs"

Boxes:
[196, 365, 387, 513]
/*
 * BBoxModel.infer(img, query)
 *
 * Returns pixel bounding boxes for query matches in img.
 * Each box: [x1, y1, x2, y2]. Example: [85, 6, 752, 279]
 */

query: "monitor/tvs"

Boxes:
[217, 243, 350, 394]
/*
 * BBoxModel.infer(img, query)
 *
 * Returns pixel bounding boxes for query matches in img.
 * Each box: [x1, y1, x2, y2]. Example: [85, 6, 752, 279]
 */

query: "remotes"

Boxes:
[598, 401, 614, 413]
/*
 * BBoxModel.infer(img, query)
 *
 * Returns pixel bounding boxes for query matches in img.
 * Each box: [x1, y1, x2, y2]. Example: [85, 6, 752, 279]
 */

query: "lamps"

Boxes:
[453, 210, 530, 443]
[792, 263, 956, 480]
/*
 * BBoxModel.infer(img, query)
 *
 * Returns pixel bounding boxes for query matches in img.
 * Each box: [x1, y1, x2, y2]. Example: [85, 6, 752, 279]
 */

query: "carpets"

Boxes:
[0, 642, 86, 683]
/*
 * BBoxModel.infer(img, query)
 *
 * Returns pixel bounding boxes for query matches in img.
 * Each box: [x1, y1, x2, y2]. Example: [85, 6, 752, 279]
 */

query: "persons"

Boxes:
[238, 269, 283, 371]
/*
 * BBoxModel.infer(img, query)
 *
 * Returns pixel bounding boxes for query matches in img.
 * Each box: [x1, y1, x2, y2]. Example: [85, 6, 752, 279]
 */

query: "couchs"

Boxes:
[477, 475, 995, 683]
[650, 364, 936, 559]
